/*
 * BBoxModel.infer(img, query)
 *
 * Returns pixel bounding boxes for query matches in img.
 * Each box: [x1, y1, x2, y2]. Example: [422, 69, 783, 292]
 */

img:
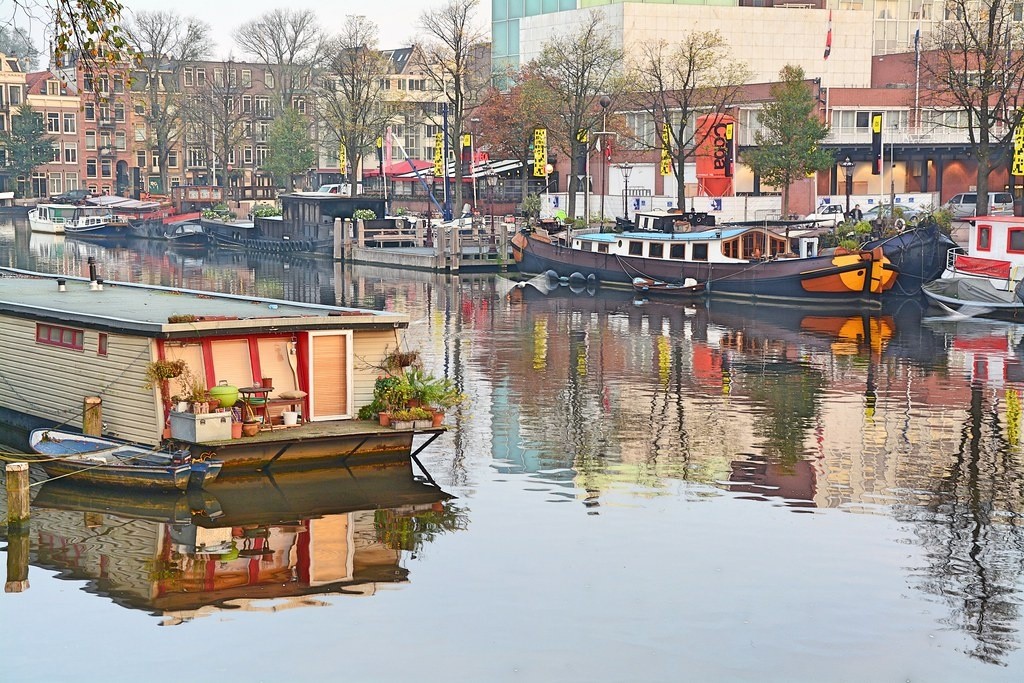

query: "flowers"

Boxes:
[355, 373, 401, 421]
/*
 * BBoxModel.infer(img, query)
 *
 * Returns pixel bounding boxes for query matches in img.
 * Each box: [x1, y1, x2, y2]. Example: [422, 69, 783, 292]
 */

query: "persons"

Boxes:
[850, 204, 863, 224]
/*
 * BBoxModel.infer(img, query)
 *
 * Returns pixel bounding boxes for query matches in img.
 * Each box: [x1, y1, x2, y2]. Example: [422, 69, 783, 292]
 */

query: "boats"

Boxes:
[26, 194, 208, 245]
[28, 427, 221, 491]
[0, 191, 36, 215]
[30, 478, 225, 525]
[922, 215, 1024, 313]
[199, 192, 412, 254]
[511, 205, 957, 302]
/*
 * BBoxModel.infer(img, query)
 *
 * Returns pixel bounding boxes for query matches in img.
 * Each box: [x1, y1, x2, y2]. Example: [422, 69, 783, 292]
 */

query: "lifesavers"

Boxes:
[263, 240, 268, 250]
[276, 242, 280, 252]
[280, 242, 285, 252]
[251, 239, 256, 247]
[272, 241, 276, 250]
[294, 241, 299, 250]
[254, 239, 259, 249]
[299, 240, 304, 250]
[305, 241, 312, 252]
[247, 239, 252, 247]
[291, 241, 295, 251]
[268, 241, 272, 251]
[285, 241, 290, 251]
[258, 240, 262, 250]
[895, 218, 905, 232]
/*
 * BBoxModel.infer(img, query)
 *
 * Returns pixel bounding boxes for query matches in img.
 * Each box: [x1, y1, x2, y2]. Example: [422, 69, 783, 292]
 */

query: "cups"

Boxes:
[262, 378, 272, 388]
[253, 381, 260, 388]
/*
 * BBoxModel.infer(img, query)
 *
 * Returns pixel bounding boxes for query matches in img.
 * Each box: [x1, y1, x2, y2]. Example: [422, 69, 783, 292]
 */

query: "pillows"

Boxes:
[278, 390, 307, 400]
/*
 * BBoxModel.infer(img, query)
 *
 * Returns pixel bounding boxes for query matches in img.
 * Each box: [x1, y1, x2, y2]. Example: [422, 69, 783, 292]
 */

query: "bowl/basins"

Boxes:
[209, 380, 238, 407]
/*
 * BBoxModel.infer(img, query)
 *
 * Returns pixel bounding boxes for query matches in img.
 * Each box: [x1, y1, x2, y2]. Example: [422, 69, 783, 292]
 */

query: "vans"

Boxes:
[318, 184, 364, 196]
[942, 191, 1013, 220]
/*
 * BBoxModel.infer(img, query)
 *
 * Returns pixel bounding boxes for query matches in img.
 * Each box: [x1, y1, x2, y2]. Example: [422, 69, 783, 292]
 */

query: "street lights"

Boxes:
[620, 161, 634, 220]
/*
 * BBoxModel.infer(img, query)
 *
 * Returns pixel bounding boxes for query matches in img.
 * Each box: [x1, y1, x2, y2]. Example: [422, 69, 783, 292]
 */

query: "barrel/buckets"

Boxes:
[282, 412, 297, 425]
[231, 422, 242, 439]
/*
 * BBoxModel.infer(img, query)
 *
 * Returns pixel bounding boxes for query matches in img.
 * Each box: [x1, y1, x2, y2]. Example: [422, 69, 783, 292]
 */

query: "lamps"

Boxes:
[290, 333, 298, 355]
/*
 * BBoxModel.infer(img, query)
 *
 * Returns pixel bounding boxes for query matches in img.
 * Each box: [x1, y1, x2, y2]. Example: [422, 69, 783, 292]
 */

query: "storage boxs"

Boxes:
[170, 410, 233, 443]
[178, 525, 233, 554]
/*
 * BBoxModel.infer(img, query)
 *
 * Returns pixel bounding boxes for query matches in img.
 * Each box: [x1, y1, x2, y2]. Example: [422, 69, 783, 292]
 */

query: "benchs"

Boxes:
[249, 398, 306, 426]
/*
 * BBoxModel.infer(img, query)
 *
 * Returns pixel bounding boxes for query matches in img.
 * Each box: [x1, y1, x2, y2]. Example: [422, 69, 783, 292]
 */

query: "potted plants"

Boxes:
[141, 358, 222, 413]
[202, 203, 237, 222]
[353, 344, 475, 430]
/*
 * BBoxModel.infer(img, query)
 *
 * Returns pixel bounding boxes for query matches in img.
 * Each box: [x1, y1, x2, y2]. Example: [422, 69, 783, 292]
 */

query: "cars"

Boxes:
[803, 205, 844, 229]
[49, 190, 92, 203]
[860, 204, 923, 222]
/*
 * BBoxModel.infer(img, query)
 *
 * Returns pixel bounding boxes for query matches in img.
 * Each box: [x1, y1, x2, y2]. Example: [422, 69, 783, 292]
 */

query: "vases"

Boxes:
[233, 421, 261, 439]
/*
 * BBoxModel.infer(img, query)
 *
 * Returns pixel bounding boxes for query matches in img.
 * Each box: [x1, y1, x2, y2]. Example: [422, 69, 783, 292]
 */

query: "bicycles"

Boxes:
[519, 216, 541, 227]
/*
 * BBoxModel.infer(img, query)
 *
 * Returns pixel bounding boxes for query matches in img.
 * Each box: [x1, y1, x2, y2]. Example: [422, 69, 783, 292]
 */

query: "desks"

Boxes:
[238, 525, 275, 556]
[237, 386, 275, 432]
